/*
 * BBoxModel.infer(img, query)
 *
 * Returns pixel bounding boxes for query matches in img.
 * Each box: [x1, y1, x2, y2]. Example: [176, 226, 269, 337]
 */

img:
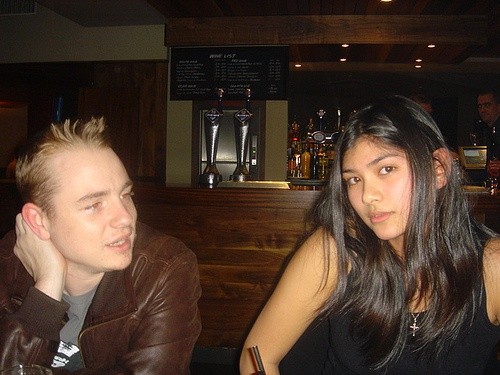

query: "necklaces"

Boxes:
[407, 281, 436, 337]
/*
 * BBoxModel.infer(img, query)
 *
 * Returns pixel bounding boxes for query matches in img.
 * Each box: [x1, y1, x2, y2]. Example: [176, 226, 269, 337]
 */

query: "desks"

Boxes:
[167, 181, 499, 344]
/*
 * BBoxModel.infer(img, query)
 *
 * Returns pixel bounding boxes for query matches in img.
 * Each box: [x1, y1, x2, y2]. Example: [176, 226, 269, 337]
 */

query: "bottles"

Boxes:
[287, 138, 327, 180]
[490, 176, 498, 197]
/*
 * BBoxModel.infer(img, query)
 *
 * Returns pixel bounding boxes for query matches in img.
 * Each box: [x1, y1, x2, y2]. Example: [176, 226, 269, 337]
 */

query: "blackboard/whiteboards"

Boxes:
[170, 47, 288, 100]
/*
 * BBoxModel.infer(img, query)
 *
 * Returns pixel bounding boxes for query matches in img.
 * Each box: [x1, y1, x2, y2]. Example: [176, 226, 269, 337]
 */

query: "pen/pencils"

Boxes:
[250, 345, 267, 375]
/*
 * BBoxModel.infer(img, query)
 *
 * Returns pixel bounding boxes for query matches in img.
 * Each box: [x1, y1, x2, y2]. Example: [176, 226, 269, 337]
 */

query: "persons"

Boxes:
[409, 88, 500, 187]
[0, 116, 201, 375]
[239, 95, 500, 375]
